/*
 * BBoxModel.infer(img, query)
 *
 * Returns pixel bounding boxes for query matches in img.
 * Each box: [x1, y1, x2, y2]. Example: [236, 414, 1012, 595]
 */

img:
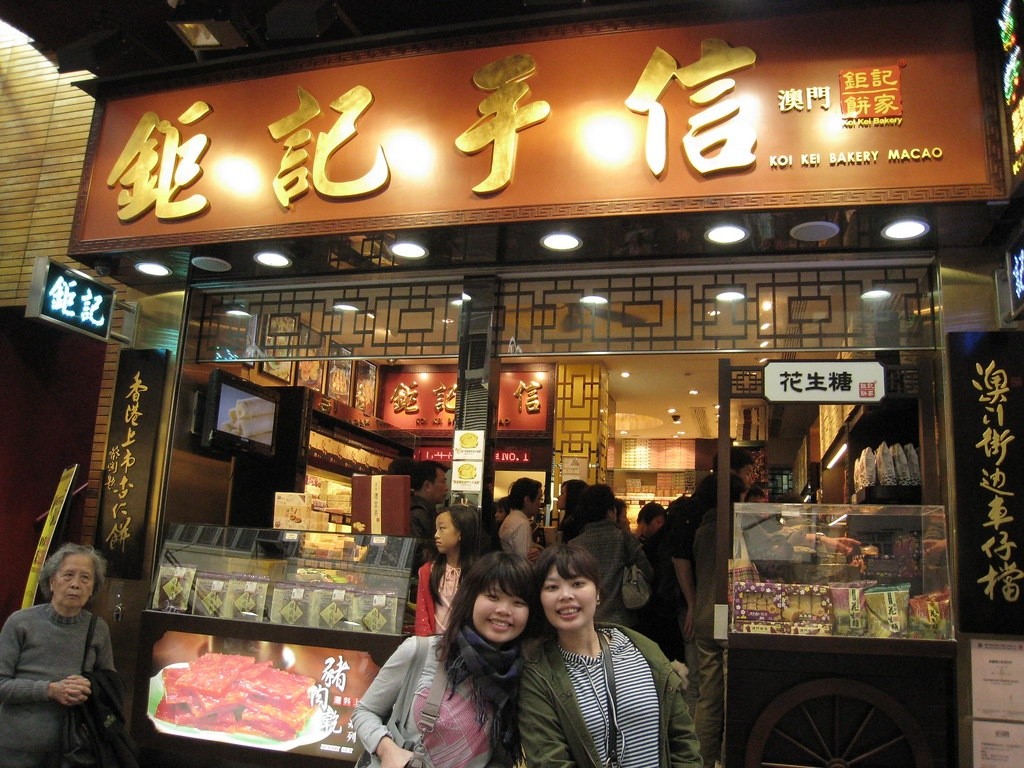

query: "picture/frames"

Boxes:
[245, 310, 377, 416]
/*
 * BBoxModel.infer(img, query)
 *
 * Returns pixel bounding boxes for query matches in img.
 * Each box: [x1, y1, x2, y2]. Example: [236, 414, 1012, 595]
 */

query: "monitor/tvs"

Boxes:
[200, 367, 280, 458]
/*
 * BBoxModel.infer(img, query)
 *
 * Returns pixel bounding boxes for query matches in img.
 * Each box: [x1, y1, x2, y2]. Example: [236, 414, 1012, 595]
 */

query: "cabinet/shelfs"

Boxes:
[819, 363, 922, 505]
[613, 468, 695, 533]
[263, 386, 416, 526]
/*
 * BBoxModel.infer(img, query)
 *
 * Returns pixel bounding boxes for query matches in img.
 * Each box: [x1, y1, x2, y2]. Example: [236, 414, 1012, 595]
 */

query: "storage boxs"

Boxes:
[736, 406, 766, 441]
[305, 425, 411, 537]
[615, 439, 695, 530]
[818, 310, 920, 458]
[274, 492, 329, 532]
[734, 582, 835, 636]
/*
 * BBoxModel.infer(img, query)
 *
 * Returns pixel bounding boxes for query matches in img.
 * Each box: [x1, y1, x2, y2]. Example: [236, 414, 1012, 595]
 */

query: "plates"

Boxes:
[145, 662, 338, 751]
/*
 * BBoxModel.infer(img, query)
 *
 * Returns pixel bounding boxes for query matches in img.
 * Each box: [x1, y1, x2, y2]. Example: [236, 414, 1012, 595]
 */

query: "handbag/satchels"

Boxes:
[61, 613, 102, 768]
[355, 636, 453, 768]
[622, 531, 651, 610]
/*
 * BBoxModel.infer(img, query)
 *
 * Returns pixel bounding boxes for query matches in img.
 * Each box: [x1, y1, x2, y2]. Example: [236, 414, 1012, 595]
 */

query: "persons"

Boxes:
[480, 477, 545, 571]
[365, 457, 448, 602]
[553, 479, 680, 665]
[0, 543, 118, 768]
[665, 443, 862, 768]
[348, 552, 552, 768]
[415, 504, 482, 636]
[517, 543, 705, 768]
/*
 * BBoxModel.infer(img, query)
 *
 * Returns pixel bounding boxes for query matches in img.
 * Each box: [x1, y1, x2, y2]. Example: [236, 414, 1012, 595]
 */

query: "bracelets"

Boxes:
[815, 532, 825, 546]
